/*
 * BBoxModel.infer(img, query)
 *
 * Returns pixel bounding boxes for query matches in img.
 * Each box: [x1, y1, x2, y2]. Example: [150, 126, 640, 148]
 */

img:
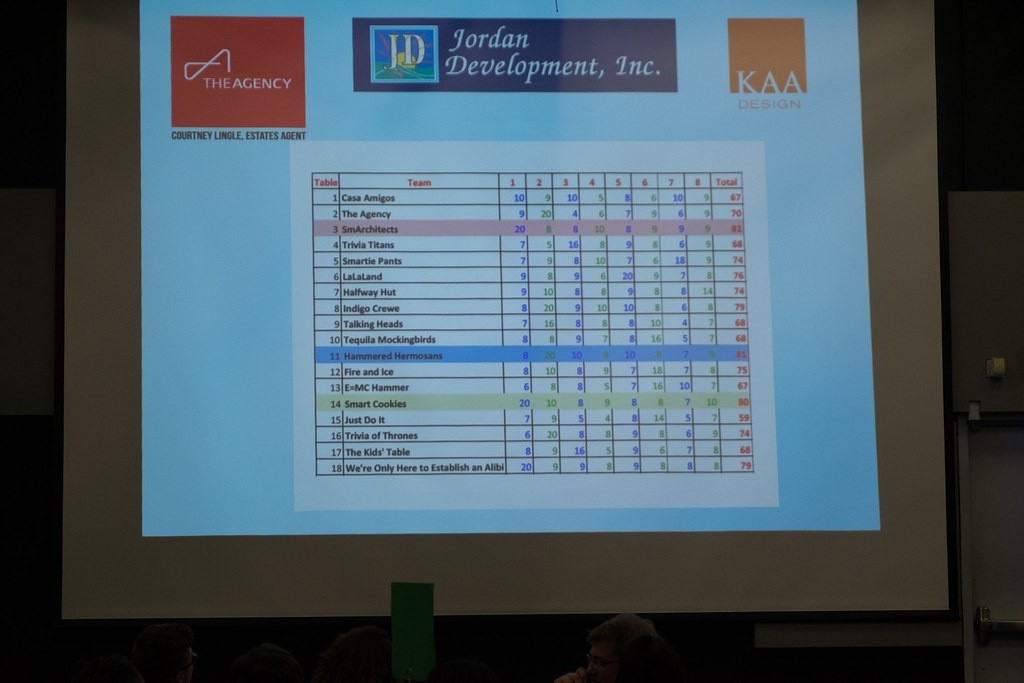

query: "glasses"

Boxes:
[586, 653, 618, 670]
[182, 653, 198, 665]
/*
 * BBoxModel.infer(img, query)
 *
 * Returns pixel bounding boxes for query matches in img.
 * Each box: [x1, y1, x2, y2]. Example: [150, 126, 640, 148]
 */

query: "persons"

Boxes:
[554, 613, 657, 683]
[71, 625, 502, 683]
